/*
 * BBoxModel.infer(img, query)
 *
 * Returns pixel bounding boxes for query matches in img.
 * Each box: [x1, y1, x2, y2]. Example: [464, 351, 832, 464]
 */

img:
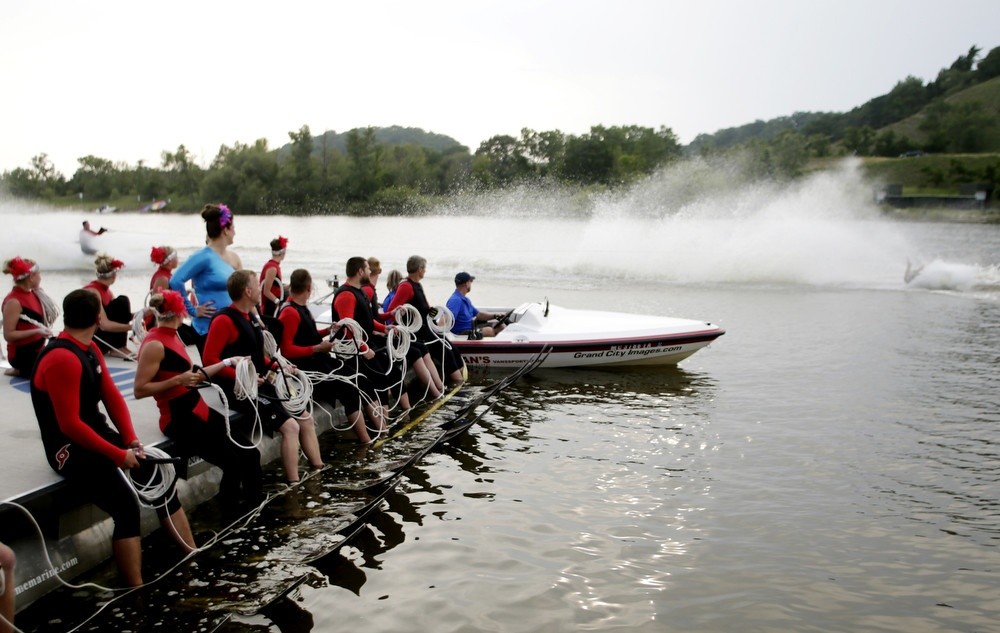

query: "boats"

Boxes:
[438, 294, 729, 371]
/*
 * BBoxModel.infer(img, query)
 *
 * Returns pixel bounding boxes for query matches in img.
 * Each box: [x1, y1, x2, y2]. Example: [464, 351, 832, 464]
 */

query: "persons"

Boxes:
[436, 272, 504, 340]
[278, 255, 465, 443]
[0, 543, 15, 633]
[30, 289, 198, 586]
[133, 270, 324, 512]
[0, 203, 289, 377]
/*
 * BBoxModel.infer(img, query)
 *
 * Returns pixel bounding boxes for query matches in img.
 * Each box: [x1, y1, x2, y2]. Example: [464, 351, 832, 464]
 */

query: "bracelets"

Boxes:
[223, 359, 231, 367]
[274, 298, 279, 304]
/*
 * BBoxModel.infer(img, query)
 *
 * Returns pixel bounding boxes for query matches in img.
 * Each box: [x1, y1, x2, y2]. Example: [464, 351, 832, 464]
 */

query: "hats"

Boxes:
[455, 272, 475, 283]
